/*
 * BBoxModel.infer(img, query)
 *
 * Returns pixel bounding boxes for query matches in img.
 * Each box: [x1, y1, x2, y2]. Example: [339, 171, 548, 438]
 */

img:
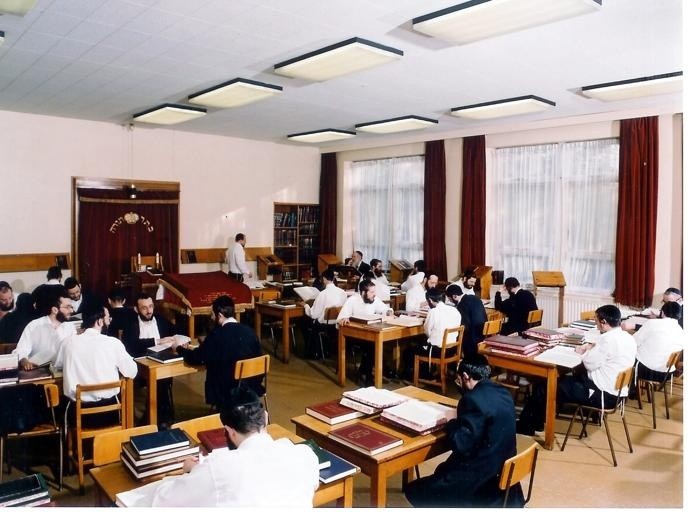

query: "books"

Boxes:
[293, 286, 321, 307]
[0, 473, 51, 506]
[339, 397, 383, 415]
[196, 428, 237, 452]
[275, 229, 297, 246]
[534, 344, 583, 369]
[571, 319, 597, 328]
[348, 316, 383, 326]
[343, 387, 409, 409]
[114, 477, 160, 506]
[119, 426, 203, 482]
[0, 354, 63, 388]
[299, 224, 318, 235]
[522, 325, 564, 351]
[326, 421, 404, 457]
[386, 310, 428, 328]
[300, 238, 313, 262]
[147, 269, 162, 275]
[313, 446, 361, 485]
[254, 277, 304, 288]
[291, 438, 332, 471]
[299, 206, 320, 223]
[622, 316, 649, 330]
[381, 399, 457, 432]
[481, 298, 492, 308]
[380, 401, 459, 436]
[146, 334, 191, 364]
[305, 398, 364, 426]
[261, 297, 297, 308]
[570, 324, 594, 331]
[273, 211, 297, 227]
[390, 289, 407, 296]
[483, 326, 540, 358]
[566, 334, 586, 345]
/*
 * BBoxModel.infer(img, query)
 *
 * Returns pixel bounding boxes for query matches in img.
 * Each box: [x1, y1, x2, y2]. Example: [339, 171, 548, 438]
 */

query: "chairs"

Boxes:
[304, 306, 342, 364]
[210, 354, 271, 425]
[561, 367, 635, 467]
[0, 383, 63, 493]
[499, 441, 541, 507]
[527, 308, 544, 328]
[0, 343, 19, 354]
[413, 324, 465, 396]
[665, 373, 683, 399]
[334, 344, 362, 376]
[383, 299, 396, 312]
[259, 290, 297, 359]
[482, 319, 502, 338]
[580, 310, 596, 321]
[92, 424, 158, 468]
[63, 376, 127, 496]
[637, 351, 681, 429]
[170, 413, 225, 444]
[488, 313, 506, 323]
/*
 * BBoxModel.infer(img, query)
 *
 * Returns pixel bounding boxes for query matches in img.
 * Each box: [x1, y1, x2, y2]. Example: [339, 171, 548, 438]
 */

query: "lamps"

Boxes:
[131, 102, 208, 128]
[286, 128, 359, 144]
[353, 115, 441, 136]
[580, 70, 686, 103]
[273, 36, 405, 84]
[411, 0, 605, 47]
[451, 95, 557, 121]
[188, 78, 284, 110]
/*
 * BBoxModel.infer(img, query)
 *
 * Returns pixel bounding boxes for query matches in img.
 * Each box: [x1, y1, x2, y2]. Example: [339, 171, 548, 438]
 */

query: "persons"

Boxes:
[225, 233, 253, 283]
[0, 281, 19, 320]
[0, 292, 42, 344]
[31, 266, 67, 316]
[335, 281, 394, 388]
[425, 272, 439, 288]
[446, 270, 477, 295]
[516, 305, 637, 436]
[401, 260, 431, 311]
[358, 258, 391, 292]
[363, 271, 391, 301]
[148, 388, 321, 507]
[51, 305, 138, 446]
[350, 251, 370, 284]
[404, 353, 526, 508]
[64, 277, 101, 316]
[401, 287, 462, 383]
[646, 288, 683, 329]
[345, 257, 353, 267]
[494, 278, 542, 338]
[297, 271, 347, 361]
[628, 300, 683, 401]
[121, 294, 190, 431]
[172, 295, 267, 405]
[103, 289, 138, 338]
[445, 285, 488, 362]
[17, 293, 77, 371]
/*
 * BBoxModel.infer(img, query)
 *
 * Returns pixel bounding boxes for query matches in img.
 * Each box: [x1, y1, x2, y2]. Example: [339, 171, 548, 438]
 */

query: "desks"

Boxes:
[338, 308, 506, 388]
[263, 278, 357, 300]
[89, 424, 361, 509]
[477, 343, 562, 451]
[255, 293, 406, 365]
[27, 376, 133, 428]
[290, 387, 457, 508]
[251, 288, 281, 297]
[136, 358, 207, 424]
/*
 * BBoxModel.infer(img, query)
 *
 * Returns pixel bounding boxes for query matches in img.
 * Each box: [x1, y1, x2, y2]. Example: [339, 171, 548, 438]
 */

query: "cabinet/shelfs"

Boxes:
[273, 202, 321, 283]
